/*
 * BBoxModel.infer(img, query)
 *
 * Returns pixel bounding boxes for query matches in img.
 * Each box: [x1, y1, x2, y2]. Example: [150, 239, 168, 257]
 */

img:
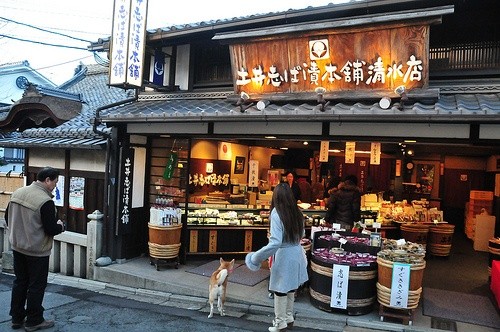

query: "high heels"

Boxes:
[269, 318, 294, 332]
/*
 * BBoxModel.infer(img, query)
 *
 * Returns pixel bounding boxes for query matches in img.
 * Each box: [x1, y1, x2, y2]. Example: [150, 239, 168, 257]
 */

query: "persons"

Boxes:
[285, 171, 302, 203]
[245, 183, 309, 332]
[325, 174, 360, 227]
[4, 167, 64, 332]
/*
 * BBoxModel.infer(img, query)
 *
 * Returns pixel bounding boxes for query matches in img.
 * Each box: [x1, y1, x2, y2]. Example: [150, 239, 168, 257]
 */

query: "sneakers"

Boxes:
[25, 320, 55, 331]
[12, 318, 25, 329]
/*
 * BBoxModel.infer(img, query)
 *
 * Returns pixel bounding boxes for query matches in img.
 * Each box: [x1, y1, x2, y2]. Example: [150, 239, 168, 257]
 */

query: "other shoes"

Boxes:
[269, 293, 274, 299]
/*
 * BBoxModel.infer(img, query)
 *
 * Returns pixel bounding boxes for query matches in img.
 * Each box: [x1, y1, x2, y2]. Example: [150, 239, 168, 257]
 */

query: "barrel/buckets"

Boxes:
[375, 249, 426, 309]
[147, 221, 183, 259]
[488, 240, 500, 281]
[428, 224, 456, 257]
[400, 222, 429, 251]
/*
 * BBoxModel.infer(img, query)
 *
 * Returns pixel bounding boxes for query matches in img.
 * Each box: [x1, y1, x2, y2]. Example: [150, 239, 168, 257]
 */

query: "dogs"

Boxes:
[207, 256, 235, 319]
